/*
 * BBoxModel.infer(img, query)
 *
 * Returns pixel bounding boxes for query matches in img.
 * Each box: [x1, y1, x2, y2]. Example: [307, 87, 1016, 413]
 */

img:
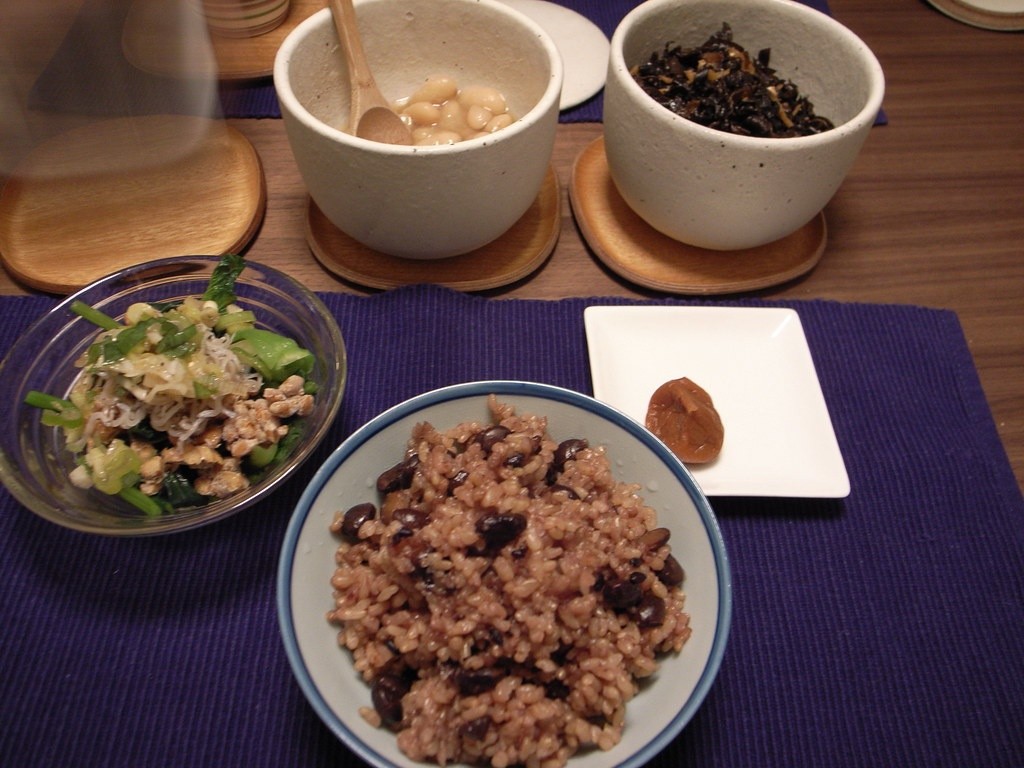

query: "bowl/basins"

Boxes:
[928, 0, 1024, 30]
[273, 0, 564, 260]
[183, 0, 289, 39]
[603, 0, 885, 252]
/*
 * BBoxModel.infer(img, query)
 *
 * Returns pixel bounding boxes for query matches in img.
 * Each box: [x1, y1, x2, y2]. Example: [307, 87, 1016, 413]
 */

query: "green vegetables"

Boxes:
[24, 253, 320, 517]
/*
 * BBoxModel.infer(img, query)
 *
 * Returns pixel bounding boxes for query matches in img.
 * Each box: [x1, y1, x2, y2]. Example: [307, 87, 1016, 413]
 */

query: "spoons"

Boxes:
[328, 0, 416, 152]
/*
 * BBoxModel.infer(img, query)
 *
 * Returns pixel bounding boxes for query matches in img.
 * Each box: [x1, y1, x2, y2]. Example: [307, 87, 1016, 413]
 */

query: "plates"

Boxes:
[0, 255, 346, 538]
[493, 0, 611, 112]
[584, 306, 850, 497]
[275, 380, 733, 768]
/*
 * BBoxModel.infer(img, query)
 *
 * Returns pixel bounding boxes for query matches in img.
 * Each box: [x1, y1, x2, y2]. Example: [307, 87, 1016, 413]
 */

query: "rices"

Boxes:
[326, 392, 694, 768]
